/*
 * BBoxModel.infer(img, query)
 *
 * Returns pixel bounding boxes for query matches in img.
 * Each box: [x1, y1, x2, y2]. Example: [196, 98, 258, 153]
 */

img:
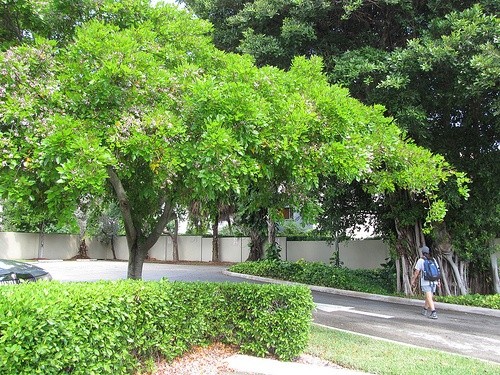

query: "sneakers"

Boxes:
[419, 309, 427, 315]
[429, 313, 437, 319]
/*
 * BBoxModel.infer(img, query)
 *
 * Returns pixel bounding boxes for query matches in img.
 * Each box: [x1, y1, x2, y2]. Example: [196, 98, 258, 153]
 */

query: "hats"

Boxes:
[419, 246, 429, 254]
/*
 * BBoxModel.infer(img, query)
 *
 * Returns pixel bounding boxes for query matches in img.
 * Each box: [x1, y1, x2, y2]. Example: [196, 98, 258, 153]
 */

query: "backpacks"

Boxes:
[422, 257, 439, 280]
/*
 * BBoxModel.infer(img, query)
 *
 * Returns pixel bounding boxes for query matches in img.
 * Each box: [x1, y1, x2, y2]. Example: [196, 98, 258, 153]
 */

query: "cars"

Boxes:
[0, 258, 53, 285]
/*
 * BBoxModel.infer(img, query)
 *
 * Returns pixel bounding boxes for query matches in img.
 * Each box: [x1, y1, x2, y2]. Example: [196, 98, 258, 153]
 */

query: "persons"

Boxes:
[411, 246, 441, 319]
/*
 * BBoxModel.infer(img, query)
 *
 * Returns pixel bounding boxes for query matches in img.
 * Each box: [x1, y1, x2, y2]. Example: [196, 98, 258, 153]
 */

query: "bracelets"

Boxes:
[437, 281, 440, 282]
